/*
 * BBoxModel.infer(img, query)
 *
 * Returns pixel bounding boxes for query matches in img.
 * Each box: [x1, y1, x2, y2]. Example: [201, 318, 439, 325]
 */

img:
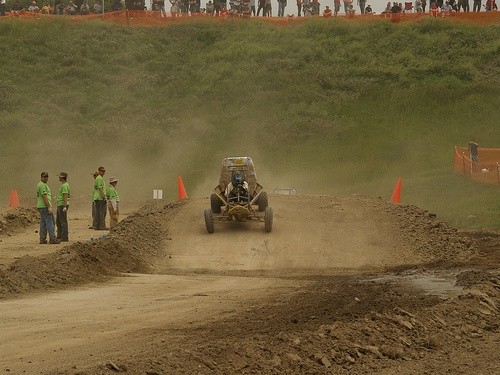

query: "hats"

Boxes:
[41, 171, 48, 178]
[57, 172, 68, 180]
[109, 176, 118, 184]
[90, 172, 99, 176]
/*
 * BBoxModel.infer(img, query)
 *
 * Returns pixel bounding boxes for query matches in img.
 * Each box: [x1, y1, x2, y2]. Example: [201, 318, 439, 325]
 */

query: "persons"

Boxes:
[1, 0, 499, 19]
[89, 172, 102, 229]
[468, 139, 478, 168]
[36, 170, 60, 244]
[94, 166, 109, 230]
[54, 171, 71, 243]
[106, 176, 120, 231]
[223, 171, 248, 197]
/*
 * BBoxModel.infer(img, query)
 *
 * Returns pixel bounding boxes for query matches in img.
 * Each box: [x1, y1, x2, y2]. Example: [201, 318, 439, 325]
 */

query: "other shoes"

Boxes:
[57, 236, 68, 241]
[40, 239, 47, 243]
[95, 227, 100, 230]
[49, 239, 59, 244]
[89, 226, 95, 229]
[100, 227, 109, 230]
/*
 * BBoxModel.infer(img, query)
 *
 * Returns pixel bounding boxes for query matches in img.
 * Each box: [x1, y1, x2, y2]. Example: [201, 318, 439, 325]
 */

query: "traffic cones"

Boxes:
[388, 176, 402, 205]
[177, 175, 188, 200]
[8, 188, 20, 208]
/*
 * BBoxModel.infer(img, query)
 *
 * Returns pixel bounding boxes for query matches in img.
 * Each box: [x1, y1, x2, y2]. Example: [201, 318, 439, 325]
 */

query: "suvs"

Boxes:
[272, 188, 296, 195]
